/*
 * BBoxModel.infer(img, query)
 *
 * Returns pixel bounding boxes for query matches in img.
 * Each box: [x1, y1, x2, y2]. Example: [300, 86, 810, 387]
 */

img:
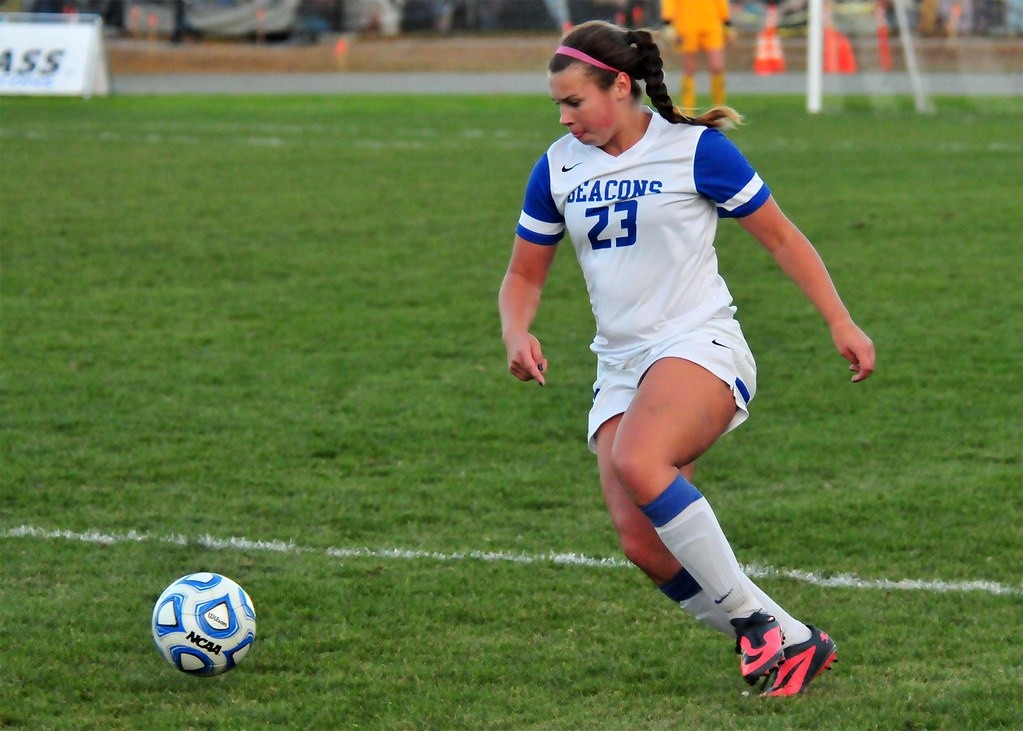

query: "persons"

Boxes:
[496, 20, 872, 701]
[660, 0, 737, 125]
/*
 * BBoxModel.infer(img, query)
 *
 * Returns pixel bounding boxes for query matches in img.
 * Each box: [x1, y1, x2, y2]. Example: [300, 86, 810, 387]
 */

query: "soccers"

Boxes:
[150, 572, 257, 678]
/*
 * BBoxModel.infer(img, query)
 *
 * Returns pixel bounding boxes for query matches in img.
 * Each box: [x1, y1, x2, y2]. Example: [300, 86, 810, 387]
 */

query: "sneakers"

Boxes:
[757, 624, 838, 698]
[729, 611, 784, 686]
